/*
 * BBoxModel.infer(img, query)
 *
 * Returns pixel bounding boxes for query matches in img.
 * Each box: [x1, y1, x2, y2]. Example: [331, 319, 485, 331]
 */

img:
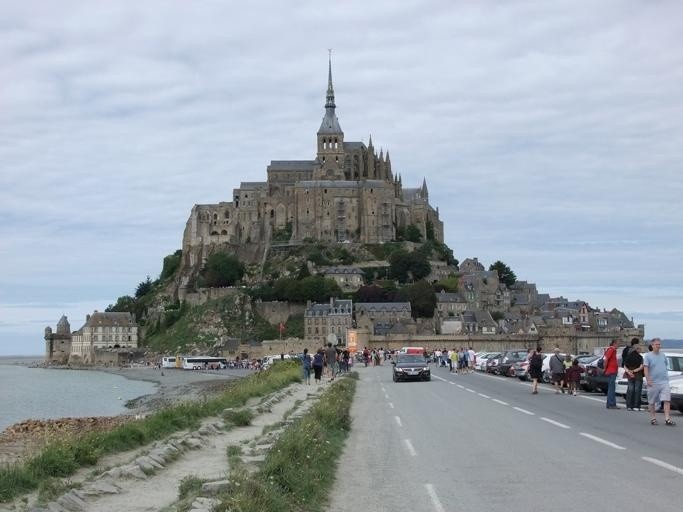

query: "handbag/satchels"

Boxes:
[596, 358, 604, 369]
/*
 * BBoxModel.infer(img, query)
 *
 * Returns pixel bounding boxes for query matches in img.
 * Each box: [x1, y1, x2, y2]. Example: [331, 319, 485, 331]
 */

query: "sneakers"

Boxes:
[606, 405, 648, 411]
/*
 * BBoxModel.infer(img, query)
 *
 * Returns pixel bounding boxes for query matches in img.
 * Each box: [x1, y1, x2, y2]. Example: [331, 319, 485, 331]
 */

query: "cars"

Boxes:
[499, 342, 682, 414]
[388, 353, 430, 382]
[384, 343, 499, 373]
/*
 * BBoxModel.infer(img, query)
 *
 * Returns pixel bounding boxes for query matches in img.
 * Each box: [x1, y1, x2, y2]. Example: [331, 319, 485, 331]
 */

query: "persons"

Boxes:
[567, 359, 584, 398]
[563, 355, 572, 394]
[549, 348, 565, 394]
[603, 340, 621, 409]
[621, 338, 639, 368]
[203, 342, 476, 384]
[648, 344, 662, 411]
[624, 344, 647, 411]
[644, 338, 677, 425]
[529, 347, 546, 393]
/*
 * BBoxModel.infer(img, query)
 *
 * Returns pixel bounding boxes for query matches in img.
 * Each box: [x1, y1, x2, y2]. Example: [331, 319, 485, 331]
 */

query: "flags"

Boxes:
[280, 323, 285, 334]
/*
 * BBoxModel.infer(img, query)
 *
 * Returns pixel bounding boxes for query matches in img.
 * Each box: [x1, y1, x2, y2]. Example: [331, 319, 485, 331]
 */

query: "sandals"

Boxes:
[665, 418, 675, 425]
[650, 418, 658, 424]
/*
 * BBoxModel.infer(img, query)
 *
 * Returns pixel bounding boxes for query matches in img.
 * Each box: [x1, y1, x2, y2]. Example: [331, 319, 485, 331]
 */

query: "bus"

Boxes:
[156, 346, 367, 372]
